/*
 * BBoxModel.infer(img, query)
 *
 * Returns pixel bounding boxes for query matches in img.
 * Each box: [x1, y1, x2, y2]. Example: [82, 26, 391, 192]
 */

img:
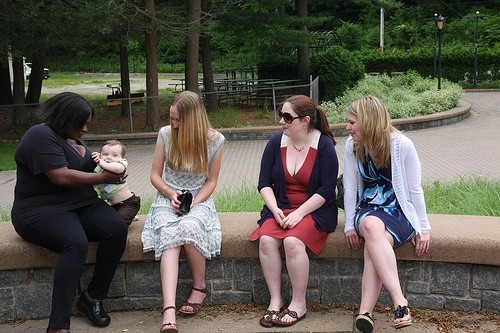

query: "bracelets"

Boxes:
[163, 186, 168, 196]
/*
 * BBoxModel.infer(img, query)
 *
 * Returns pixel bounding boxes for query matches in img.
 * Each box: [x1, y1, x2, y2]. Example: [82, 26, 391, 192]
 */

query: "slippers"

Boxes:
[272, 309, 306, 327]
[259, 305, 285, 328]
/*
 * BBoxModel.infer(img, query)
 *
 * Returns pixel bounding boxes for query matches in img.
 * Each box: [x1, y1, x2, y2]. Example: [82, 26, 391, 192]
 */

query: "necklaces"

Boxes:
[291, 137, 304, 151]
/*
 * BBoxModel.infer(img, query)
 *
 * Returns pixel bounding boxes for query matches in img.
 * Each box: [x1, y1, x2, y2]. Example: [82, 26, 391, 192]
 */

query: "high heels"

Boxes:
[46, 327, 71, 333]
[75, 291, 111, 328]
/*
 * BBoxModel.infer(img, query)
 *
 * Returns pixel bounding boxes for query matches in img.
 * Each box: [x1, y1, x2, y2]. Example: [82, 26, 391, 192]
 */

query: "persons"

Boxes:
[250, 95, 339, 328]
[142, 91, 225, 333]
[344, 97, 431, 333]
[91, 140, 141, 227]
[11, 91, 128, 333]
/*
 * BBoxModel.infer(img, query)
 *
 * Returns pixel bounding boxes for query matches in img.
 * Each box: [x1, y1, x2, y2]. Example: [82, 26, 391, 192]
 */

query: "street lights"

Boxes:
[434, 13, 446, 91]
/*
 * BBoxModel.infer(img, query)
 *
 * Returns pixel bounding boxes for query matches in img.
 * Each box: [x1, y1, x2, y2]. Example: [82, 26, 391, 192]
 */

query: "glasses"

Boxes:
[277, 110, 313, 124]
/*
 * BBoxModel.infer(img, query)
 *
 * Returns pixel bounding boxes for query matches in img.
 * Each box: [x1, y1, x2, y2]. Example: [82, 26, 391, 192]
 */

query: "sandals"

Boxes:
[160, 306, 178, 333]
[355, 312, 374, 333]
[179, 286, 209, 314]
[392, 305, 412, 329]
